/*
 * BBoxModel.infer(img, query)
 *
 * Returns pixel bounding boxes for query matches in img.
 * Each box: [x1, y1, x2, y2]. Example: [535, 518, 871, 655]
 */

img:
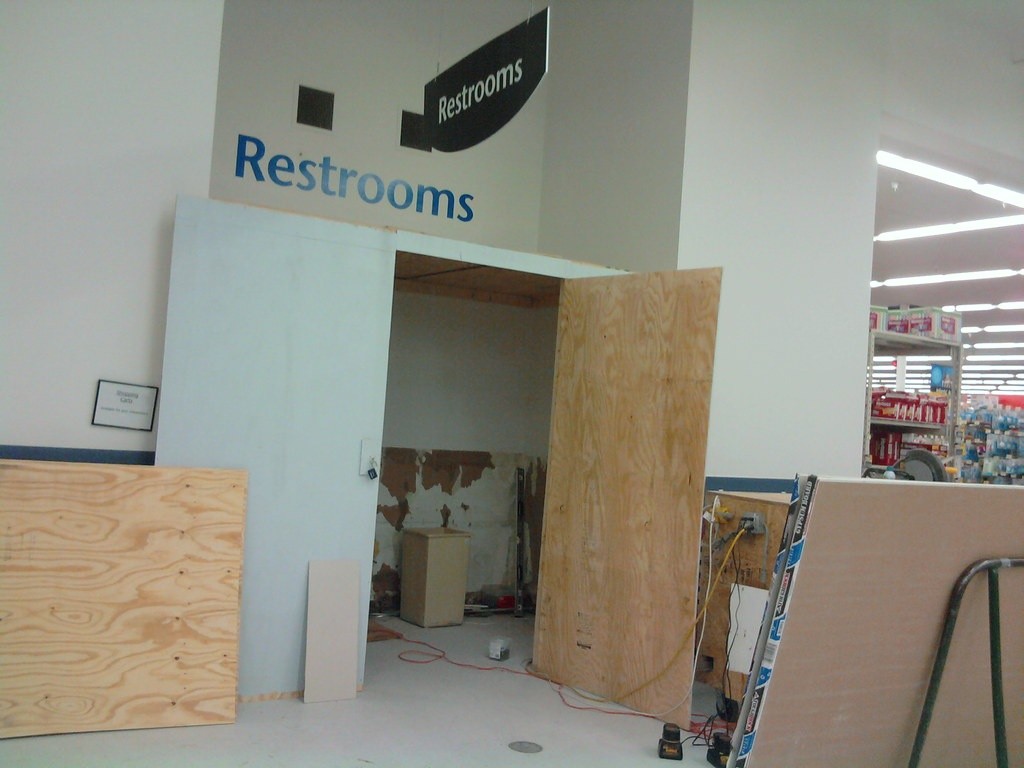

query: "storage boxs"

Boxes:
[867, 304, 962, 343]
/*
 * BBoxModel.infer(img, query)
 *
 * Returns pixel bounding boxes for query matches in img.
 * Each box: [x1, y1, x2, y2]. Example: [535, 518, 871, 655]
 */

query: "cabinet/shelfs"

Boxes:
[861, 329, 962, 483]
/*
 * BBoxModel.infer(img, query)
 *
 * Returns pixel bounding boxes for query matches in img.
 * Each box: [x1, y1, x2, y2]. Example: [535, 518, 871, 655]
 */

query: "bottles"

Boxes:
[914, 434, 945, 445]
[883, 466, 896, 480]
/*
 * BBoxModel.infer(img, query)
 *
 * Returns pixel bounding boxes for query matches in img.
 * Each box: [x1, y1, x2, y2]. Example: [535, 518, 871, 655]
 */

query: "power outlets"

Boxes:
[708, 506, 729, 525]
[742, 512, 765, 536]
